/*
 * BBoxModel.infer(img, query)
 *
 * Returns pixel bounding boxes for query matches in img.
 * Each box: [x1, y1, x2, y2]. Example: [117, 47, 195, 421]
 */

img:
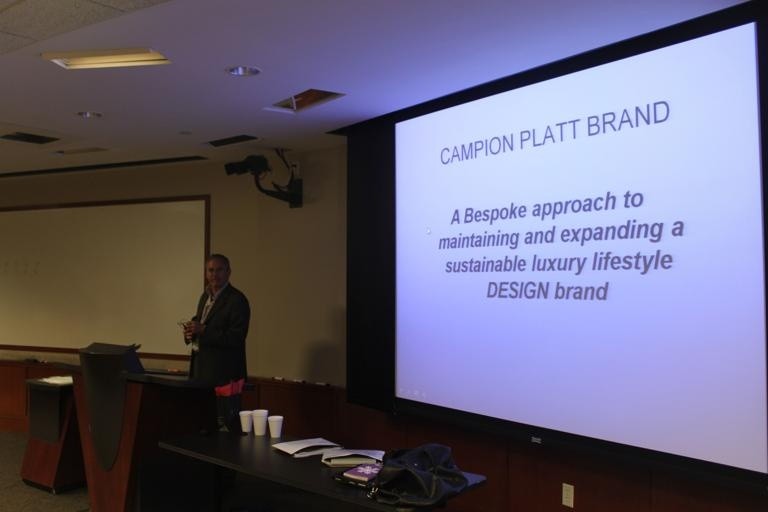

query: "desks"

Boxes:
[20, 376, 87, 493]
[157, 417, 484, 512]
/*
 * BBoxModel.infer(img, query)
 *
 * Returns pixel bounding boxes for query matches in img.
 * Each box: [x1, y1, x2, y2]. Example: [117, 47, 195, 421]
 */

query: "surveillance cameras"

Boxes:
[225, 155, 272, 176]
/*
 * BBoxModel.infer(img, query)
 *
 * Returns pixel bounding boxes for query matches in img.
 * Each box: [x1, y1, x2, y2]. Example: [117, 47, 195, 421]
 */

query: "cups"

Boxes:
[267, 415, 284, 437]
[252, 410, 268, 436]
[239, 410, 252, 433]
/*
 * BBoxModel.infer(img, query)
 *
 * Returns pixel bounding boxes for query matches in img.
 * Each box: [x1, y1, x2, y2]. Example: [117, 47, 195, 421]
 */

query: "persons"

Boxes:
[183, 253, 251, 491]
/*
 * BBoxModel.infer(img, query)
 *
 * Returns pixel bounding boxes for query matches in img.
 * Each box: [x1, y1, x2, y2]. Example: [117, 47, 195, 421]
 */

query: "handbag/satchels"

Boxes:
[365, 443, 468, 506]
[216, 395, 241, 432]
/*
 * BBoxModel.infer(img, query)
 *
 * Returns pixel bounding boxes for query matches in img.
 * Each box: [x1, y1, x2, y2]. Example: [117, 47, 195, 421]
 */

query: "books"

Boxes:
[343, 463, 383, 482]
[330, 455, 377, 466]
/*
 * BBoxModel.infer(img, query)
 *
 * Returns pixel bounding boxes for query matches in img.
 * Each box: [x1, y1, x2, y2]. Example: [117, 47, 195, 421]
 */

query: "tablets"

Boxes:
[333, 459, 383, 489]
